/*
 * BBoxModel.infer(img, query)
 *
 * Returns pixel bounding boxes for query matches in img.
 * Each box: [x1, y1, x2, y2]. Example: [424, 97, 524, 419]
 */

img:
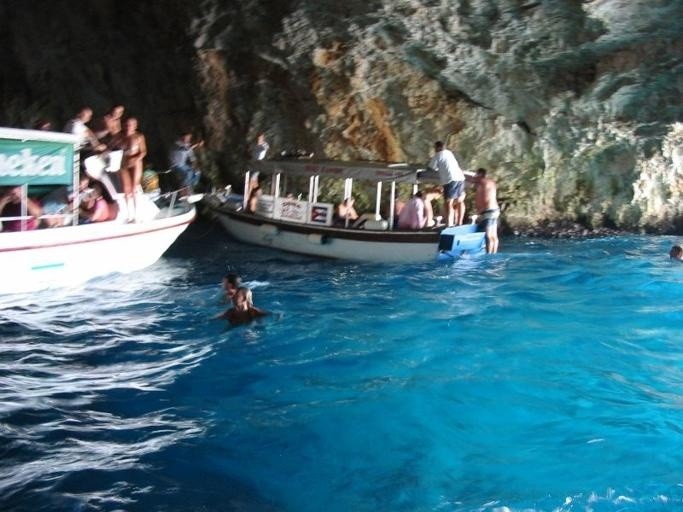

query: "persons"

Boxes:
[209, 286, 270, 325]
[335, 142, 466, 230]
[246, 133, 268, 202]
[669, 244, 682, 262]
[212, 271, 242, 303]
[463, 167, 501, 255]
[1, 102, 147, 231]
[246, 185, 263, 214]
[167, 130, 204, 197]
